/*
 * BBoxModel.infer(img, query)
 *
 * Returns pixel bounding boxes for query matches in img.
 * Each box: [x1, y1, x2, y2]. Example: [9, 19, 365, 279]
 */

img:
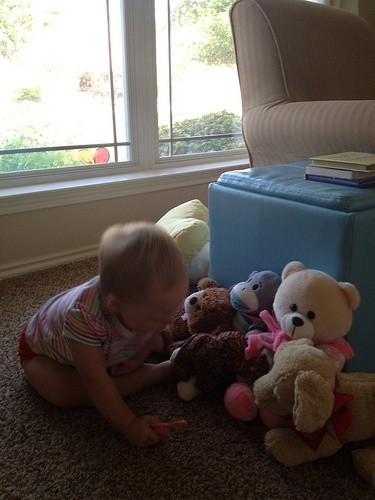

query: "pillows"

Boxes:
[155, 199, 209, 285]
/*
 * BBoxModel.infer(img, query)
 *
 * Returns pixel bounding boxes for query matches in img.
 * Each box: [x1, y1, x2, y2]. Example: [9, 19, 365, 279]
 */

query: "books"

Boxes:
[304, 151, 375, 189]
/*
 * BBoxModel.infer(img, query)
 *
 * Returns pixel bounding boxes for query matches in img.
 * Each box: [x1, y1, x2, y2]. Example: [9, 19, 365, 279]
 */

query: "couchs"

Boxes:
[230, 0, 375, 167]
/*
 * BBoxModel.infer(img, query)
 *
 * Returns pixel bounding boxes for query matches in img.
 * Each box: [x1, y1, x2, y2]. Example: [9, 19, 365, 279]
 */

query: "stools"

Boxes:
[207, 159, 375, 372]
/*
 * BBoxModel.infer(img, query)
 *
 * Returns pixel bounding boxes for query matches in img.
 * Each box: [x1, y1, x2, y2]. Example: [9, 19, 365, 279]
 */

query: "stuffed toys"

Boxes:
[152, 199, 375, 486]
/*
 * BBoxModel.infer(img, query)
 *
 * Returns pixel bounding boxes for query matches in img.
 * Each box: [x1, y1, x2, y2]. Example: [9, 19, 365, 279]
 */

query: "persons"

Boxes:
[18, 221, 191, 448]
